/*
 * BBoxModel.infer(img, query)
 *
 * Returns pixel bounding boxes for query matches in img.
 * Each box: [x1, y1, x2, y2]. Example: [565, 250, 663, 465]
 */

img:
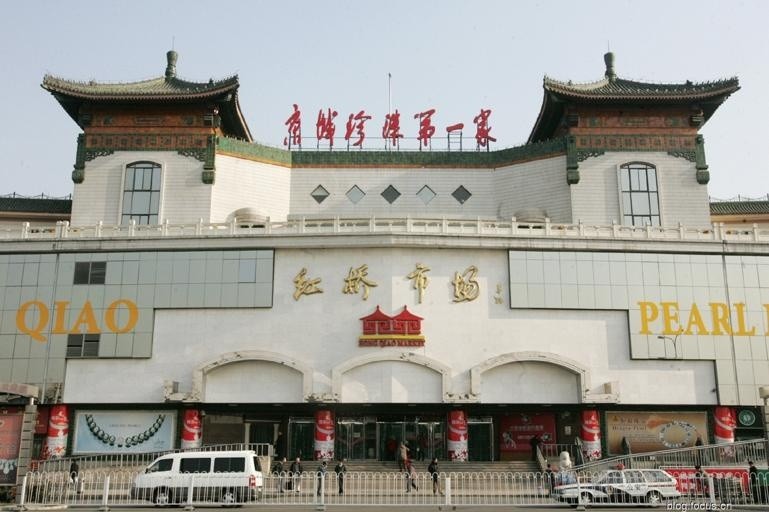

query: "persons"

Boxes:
[403, 459, 419, 493]
[272, 456, 288, 493]
[315, 461, 329, 497]
[273, 430, 288, 461]
[694, 465, 710, 498]
[529, 434, 541, 461]
[749, 460, 758, 486]
[334, 458, 347, 496]
[289, 456, 304, 492]
[544, 463, 554, 482]
[415, 431, 426, 462]
[69, 458, 79, 484]
[427, 456, 446, 496]
[694, 436, 704, 457]
[621, 436, 631, 465]
[397, 440, 411, 472]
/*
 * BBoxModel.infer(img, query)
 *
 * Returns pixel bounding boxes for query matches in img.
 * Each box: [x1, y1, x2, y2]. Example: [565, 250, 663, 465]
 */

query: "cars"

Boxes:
[551, 464, 681, 508]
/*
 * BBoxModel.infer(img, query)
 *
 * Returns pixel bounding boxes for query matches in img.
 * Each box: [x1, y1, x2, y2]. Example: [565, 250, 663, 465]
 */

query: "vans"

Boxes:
[131, 450, 263, 508]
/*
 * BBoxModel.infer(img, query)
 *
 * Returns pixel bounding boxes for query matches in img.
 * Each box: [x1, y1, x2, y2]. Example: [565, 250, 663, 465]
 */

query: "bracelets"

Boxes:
[646, 414, 704, 448]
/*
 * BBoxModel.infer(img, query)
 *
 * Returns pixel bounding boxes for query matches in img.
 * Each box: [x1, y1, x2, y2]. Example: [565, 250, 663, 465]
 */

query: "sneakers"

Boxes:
[403, 486, 445, 500]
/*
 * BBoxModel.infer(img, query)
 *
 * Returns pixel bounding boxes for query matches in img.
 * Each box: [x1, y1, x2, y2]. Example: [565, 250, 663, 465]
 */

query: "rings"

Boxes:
[501, 431, 519, 450]
[540, 432, 555, 454]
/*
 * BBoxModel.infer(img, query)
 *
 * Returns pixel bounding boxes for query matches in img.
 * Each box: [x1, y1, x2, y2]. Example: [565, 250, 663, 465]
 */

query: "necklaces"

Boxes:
[85, 413, 165, 447]
